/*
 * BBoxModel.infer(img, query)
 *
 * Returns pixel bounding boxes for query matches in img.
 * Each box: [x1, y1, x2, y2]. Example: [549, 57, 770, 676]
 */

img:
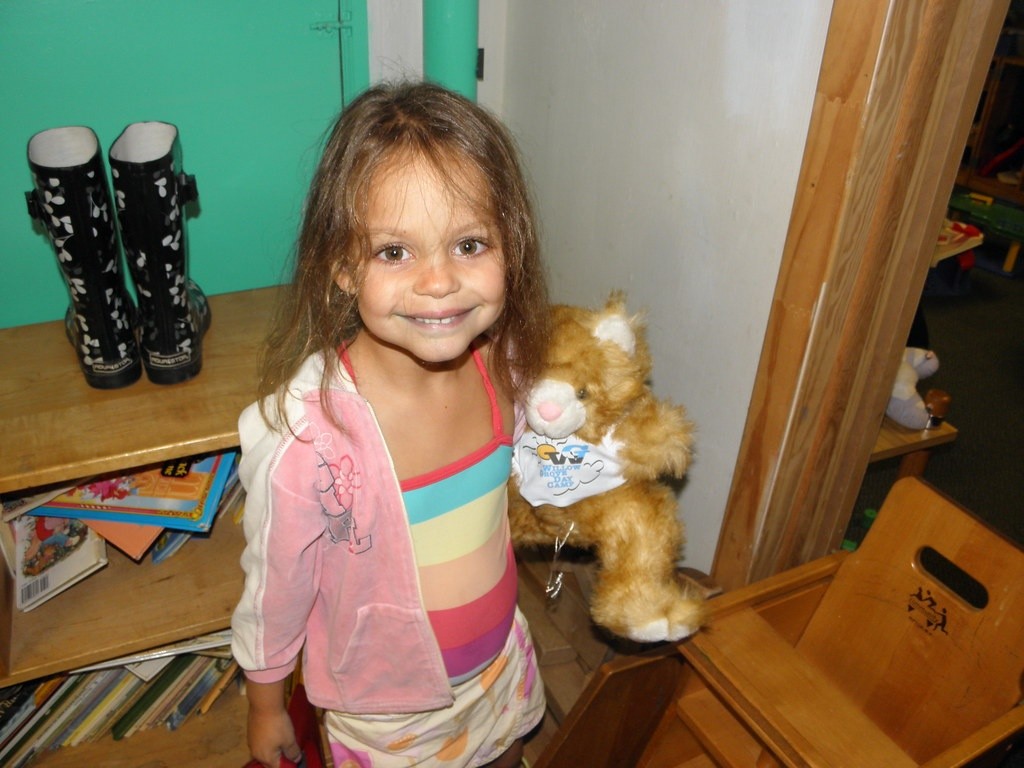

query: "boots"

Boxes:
[24, 126, 143, 390]
[108, 121, 212, 386]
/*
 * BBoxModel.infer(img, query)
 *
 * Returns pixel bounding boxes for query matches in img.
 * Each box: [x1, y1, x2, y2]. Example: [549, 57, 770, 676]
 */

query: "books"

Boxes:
[0, 448, 247, 613]
[0, 628, 246, 768]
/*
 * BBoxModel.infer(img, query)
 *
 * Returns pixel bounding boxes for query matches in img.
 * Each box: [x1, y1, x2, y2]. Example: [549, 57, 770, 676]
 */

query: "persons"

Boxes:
[232, 82, 551, 768]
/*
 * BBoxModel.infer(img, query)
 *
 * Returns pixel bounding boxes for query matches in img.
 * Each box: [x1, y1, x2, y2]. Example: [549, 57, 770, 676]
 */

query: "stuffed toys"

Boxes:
[885, 346, 939, 430]
[507, 293, 702, 642]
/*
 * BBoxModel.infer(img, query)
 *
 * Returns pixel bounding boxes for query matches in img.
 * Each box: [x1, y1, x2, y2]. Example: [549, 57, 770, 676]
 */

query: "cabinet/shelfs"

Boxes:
[0, 285, 292, 768]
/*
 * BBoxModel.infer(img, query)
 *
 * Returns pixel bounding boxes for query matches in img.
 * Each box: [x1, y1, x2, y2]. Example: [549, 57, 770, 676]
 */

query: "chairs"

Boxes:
[532, 475, 1024, 768]
